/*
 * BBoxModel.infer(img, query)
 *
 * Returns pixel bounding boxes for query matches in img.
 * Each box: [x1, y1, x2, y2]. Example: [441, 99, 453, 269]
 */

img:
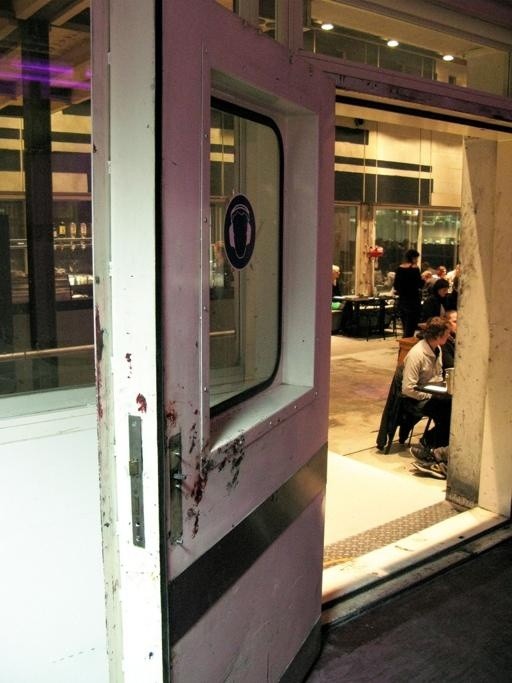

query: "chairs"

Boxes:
[331, 288, 402, 341]
[377, 361, 432, 454]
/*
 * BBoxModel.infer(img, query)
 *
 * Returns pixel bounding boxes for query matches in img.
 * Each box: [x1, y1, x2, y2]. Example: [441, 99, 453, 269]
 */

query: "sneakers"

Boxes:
[410, 446, 446, 479]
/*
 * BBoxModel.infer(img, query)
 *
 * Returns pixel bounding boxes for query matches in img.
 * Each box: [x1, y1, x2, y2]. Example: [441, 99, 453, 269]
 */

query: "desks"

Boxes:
[414, 379, 453, 456]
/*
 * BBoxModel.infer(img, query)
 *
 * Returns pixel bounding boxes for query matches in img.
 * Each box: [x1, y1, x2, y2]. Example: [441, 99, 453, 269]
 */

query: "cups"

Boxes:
[445, 367, 455, 396]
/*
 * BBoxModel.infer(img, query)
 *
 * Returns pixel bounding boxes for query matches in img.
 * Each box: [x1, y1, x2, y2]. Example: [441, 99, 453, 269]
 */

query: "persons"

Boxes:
[387, 249, 461, 478]
[332, 266, 343, 302]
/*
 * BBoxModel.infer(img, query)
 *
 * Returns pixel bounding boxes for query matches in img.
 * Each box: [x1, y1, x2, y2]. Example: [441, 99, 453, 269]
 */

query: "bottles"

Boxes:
[52, 221, 87, 238]
